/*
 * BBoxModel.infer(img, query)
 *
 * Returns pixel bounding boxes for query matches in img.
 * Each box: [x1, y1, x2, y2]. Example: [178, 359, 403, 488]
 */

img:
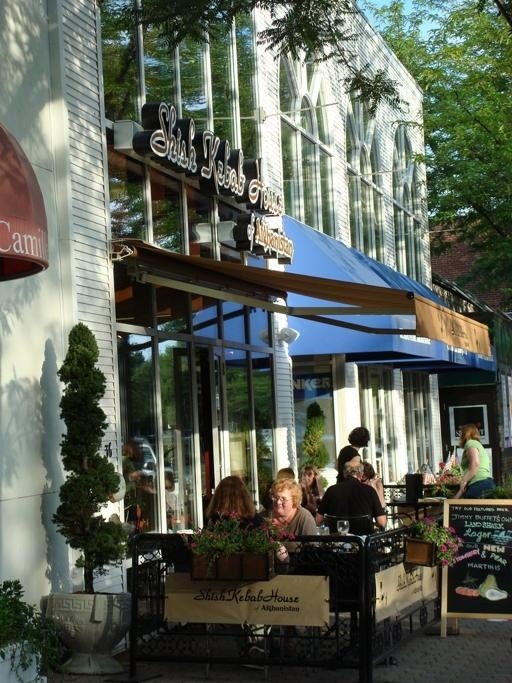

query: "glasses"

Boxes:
[272, 495, 289, 504]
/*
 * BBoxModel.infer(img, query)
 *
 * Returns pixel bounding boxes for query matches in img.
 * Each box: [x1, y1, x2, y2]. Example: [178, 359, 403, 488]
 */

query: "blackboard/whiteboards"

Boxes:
[441, 499, 512, 618]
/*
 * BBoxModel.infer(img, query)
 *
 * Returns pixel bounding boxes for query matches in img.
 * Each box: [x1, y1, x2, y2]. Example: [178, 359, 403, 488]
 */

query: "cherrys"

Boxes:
[455, 577, 480, 597]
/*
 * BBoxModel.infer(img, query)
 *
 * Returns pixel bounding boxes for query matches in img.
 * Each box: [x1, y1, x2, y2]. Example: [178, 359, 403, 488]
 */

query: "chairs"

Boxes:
[323, 512, 374, 536]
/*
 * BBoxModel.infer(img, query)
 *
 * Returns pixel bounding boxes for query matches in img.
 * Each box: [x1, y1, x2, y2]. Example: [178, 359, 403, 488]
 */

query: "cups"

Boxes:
[336, 520, 350, 534]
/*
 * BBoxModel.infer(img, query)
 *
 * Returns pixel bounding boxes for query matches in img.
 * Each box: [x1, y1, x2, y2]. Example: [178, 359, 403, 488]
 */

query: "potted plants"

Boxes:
[1, 578, 70, 681]
[46, 323, 137, 674]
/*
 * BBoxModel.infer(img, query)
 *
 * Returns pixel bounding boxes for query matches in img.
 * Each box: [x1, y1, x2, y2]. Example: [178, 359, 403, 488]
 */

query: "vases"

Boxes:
[190, 550, 276, 581]
[402, 536, 436, 568]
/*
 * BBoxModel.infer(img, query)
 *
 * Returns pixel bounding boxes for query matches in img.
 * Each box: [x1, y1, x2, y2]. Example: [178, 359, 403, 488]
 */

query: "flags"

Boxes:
[422, 454, 455, 485]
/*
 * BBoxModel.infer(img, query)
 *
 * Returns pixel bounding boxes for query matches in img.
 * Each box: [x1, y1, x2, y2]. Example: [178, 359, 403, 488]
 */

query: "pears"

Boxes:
[479, 575, 508, 602]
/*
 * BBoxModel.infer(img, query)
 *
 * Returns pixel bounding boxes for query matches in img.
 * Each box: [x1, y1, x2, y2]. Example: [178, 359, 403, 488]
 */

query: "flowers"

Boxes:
[405, 513, 465, 568]
[177, 510, 296, 562]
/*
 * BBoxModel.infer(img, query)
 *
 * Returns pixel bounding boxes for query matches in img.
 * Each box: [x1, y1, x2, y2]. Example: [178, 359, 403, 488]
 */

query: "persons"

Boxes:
[316, 461, 386, 536]
[336, 427, 370, 484]
[362, 461, 386, 508]
[122, 442, 191, 534]
[205, 465, 325, 671]
[457, 422, 497, 498]
